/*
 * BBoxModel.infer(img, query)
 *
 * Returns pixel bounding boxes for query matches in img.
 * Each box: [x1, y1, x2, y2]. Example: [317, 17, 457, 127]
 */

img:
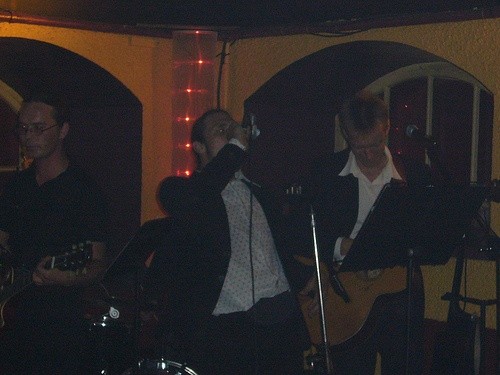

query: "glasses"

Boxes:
[16, 122, 59, 136]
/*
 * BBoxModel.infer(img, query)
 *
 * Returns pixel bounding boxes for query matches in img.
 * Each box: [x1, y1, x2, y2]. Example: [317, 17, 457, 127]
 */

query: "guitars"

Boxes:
[0, 239, 105, 329]
[290, 253, 418, 356]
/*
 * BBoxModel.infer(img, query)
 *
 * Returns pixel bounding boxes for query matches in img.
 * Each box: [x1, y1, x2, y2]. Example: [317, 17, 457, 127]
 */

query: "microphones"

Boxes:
[242, 115, 251, 128]
[405, 124, 432, 148]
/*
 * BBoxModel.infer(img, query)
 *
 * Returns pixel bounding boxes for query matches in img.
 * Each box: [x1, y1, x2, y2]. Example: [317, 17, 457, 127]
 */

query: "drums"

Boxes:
[124, 355, 202, 375]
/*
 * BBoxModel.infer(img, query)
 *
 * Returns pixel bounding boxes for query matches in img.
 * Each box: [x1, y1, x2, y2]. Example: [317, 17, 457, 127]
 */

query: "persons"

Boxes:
[175, 108, 311, 375]
[298, 94, 428, 375]
[0, 96, 109, 375]
